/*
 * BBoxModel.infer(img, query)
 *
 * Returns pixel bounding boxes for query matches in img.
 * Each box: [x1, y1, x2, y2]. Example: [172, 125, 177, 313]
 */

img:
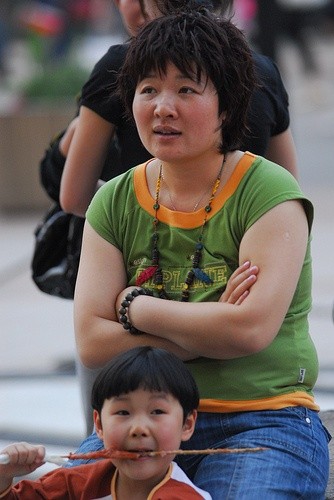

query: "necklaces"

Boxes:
[134, 153, 226, 302]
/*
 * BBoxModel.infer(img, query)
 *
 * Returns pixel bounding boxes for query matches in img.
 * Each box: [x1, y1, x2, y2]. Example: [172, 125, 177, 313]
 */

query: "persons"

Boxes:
[40, 0, 298, 218]
[0, 345, 212, 500]
[74, 5, 332, 500]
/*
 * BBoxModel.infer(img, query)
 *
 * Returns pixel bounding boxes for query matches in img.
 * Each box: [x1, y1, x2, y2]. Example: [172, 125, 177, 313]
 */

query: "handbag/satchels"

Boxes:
[31, 207, 83, 300]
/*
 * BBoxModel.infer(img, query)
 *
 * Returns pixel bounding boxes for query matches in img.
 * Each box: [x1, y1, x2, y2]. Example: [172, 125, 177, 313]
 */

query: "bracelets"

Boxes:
[119, 288, 153, 335]
